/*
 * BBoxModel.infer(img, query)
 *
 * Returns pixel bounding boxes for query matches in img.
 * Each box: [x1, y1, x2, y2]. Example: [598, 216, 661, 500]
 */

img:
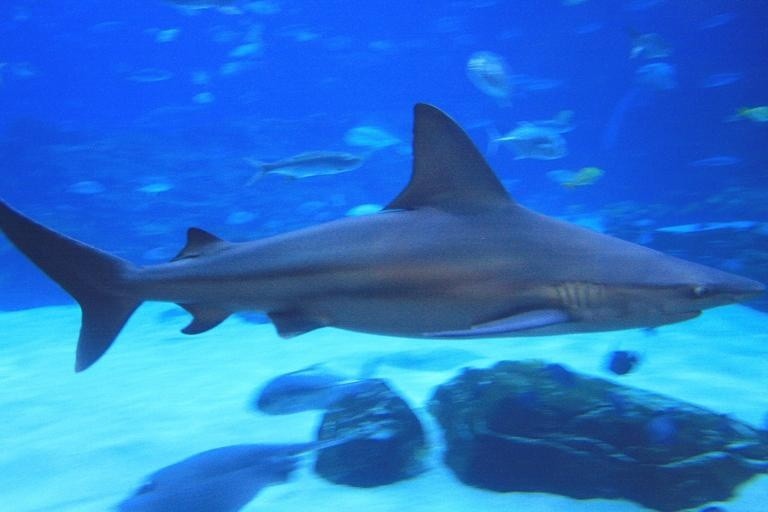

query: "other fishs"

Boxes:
[66, 0, 768, 263]
